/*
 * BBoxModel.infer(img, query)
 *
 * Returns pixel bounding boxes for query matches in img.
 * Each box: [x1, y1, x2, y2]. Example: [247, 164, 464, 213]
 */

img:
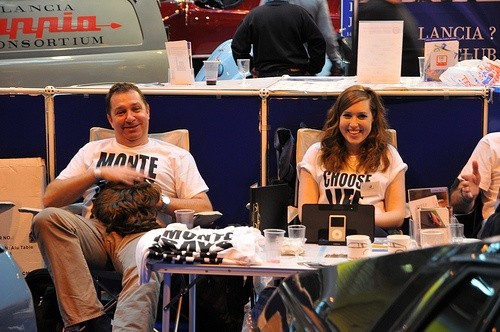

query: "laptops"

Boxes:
[302, 204, 375, 247]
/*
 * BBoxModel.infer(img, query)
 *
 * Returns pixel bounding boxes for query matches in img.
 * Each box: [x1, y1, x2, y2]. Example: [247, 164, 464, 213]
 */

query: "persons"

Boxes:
[231, 0, 345, 78]
[34, 82, 213, 332]
[450, 132, 500, 255]
[290, 84, 407, 332]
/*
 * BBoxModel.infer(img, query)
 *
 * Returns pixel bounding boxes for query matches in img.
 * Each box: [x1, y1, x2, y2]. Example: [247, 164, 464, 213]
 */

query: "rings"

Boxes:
[136, 177, 140, 181]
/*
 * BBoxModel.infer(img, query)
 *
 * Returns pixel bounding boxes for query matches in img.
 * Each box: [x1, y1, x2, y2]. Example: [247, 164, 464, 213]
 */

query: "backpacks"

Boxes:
[25, 268, 64, 332]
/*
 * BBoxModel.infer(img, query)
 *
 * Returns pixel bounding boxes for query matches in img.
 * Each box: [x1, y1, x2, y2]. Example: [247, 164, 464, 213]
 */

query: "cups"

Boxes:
[422, 228, 447, 249]
[288, 225, 306, 252]
[175, 209, 193, 228]
[204, 61, 220, 85]
[346, 235, 370, 261]
[446, 224, 464, 243]
[263, 228, 286, 262]
[418, 57, 425, 78]
[388, 235, 409, 253]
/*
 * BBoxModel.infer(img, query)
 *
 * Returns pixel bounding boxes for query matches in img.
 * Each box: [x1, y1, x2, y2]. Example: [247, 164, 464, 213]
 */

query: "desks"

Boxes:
[145, 238, 482, 332]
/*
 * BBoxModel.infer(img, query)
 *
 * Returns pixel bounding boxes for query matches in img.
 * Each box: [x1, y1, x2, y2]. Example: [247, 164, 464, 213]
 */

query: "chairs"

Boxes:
[63, 128, 190, 332]
[287, 128, 397, 234]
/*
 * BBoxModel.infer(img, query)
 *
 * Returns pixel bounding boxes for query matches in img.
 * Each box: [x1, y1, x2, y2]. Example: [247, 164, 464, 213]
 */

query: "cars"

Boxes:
[157, 0, 500, 86]
[240, 234, 500, 332]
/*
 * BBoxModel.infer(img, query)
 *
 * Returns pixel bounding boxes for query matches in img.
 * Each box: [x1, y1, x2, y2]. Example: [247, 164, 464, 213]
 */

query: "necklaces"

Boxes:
[344, 159, 366, 175]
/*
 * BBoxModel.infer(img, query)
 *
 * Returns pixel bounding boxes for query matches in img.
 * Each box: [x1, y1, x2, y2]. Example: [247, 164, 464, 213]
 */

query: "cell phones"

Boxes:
[328, 215, 346, 241]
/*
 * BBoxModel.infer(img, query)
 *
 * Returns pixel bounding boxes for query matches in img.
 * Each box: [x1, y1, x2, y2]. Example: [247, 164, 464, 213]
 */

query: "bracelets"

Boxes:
[93, 167, 102, 181]
[461, 187, 473, 203]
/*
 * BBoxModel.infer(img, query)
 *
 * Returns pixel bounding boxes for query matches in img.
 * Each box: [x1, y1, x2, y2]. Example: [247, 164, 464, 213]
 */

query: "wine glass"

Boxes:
[237, 58, 250, 81]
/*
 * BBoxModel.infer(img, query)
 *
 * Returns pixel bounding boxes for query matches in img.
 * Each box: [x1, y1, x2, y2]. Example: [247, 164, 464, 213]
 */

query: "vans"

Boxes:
[0, 0, 171, 90]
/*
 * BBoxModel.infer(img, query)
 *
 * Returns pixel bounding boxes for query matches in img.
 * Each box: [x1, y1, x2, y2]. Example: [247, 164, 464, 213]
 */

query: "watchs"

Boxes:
[159, 193, 171, 212]
